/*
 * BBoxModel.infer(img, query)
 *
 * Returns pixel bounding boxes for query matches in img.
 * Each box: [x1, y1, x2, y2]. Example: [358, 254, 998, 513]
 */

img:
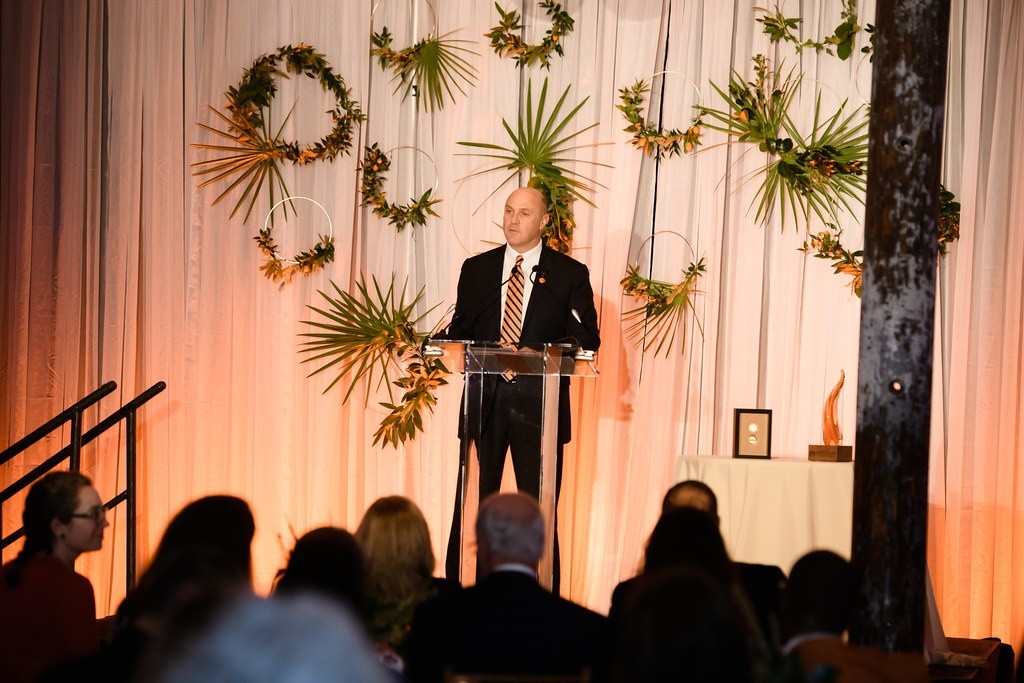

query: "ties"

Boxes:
[499, 255, 524, 382]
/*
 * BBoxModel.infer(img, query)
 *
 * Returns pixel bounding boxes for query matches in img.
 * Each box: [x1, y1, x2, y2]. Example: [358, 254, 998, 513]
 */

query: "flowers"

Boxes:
[751, 1, 861, 60]
[190, 41, 370, 227]
[483, 0, 575, 72]
[798, 223, 863, 301]
[452, 77, 617, 256]
[252, 197, 335, 291]
[855, 23, 875, 107]
[690, 53, 872, 235]
[371, 0, 481, 114]
[615, 71, 708, 165]
[621, 231, 707, 362]
[297, 271, 459, 450]
[355, 142, 443, 233]
[937, 183, 960, 259]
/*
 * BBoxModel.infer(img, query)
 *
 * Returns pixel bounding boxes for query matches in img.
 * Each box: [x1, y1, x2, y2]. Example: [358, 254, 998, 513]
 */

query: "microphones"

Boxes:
[529, 265, 580, 355]
[431, 267, 518, 339]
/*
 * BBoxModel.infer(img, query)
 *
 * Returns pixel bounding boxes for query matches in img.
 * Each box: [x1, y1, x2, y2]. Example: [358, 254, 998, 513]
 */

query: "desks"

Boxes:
[673, 454, 855, 578]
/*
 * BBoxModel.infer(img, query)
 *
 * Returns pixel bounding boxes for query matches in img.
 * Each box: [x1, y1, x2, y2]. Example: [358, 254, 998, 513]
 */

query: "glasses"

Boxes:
[70, 507, 108, 523]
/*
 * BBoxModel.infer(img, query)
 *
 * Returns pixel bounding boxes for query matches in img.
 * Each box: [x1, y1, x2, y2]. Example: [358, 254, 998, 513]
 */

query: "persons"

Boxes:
[272, 527, 409, 683]
[445, 187, 600, 598]
[607, 508, 773, 683]
[769, 549, 853, 683]
[431, 491, 616, 683]
[659, 480, 791, 665]
[105, 494, 275, 683]
[0, 471, 111, 683]
[352, 496, 456, 683]
